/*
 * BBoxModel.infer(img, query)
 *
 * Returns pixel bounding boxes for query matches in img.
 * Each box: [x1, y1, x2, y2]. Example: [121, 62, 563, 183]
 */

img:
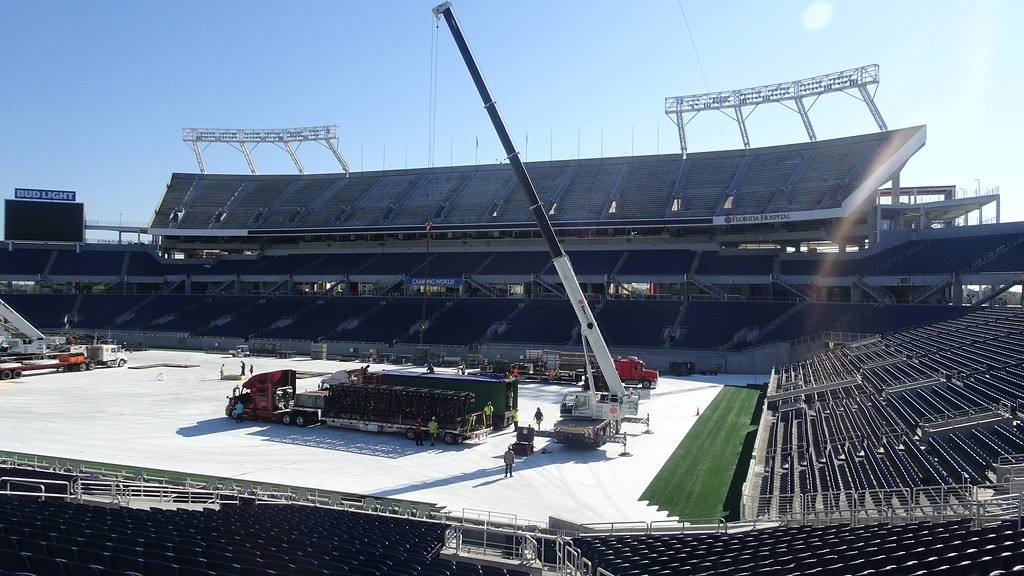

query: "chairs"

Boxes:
[0, 306, 1024, 576]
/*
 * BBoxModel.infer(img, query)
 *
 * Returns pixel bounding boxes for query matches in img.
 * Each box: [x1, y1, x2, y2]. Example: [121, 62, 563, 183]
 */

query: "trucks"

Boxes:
[228, 345, 250, 358]
[582, 356, 659, 390]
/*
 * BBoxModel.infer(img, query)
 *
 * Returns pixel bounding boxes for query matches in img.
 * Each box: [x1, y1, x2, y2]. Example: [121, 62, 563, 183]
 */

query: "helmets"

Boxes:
[487, 401, 491, 405]
[508, 445, 513, 449]
[432, 416, 436, 419]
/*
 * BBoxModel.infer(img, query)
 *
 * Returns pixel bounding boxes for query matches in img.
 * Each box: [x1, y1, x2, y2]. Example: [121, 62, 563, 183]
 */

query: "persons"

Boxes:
[504, 445, 514, 478]
[484, 401, 494, 428]
[507, 387, 514, 411]
[549, 369, 561, 385]
[415, 417, 424, 446]
[533, 407, 543, 430]
[248, 364, 253, 376]
[427, 364, 434, 373]
[234, 400, 244, 423]
[512, 408, 520, 432]
[513, 366, 519, 378]
[240, 360, 245, 376]
[364, 365, 370, 375]
[220, 364, 225, 379]
[456, 370, 460, 375]
[427, 416, 438, 446]
[461, 366, 465, 375]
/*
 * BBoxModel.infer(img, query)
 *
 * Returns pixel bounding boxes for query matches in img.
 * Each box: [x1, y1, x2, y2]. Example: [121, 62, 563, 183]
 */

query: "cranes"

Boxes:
[433, 0, 640, 420]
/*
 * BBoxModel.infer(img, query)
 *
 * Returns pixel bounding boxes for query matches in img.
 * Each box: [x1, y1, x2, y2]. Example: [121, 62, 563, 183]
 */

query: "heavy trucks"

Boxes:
[224, 365, 518, 445]
[0, 344, 128, 381]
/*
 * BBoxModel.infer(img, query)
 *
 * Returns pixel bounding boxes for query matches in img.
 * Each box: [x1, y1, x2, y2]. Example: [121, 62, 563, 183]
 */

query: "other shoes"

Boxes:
[510, 476, 513, 477]
[420, 443, 424, 446]
[416, 444, 420, 446]
[431, 444, 435, 446]
[505, 476, 507, 477]
[513, 430, 517, 432]
